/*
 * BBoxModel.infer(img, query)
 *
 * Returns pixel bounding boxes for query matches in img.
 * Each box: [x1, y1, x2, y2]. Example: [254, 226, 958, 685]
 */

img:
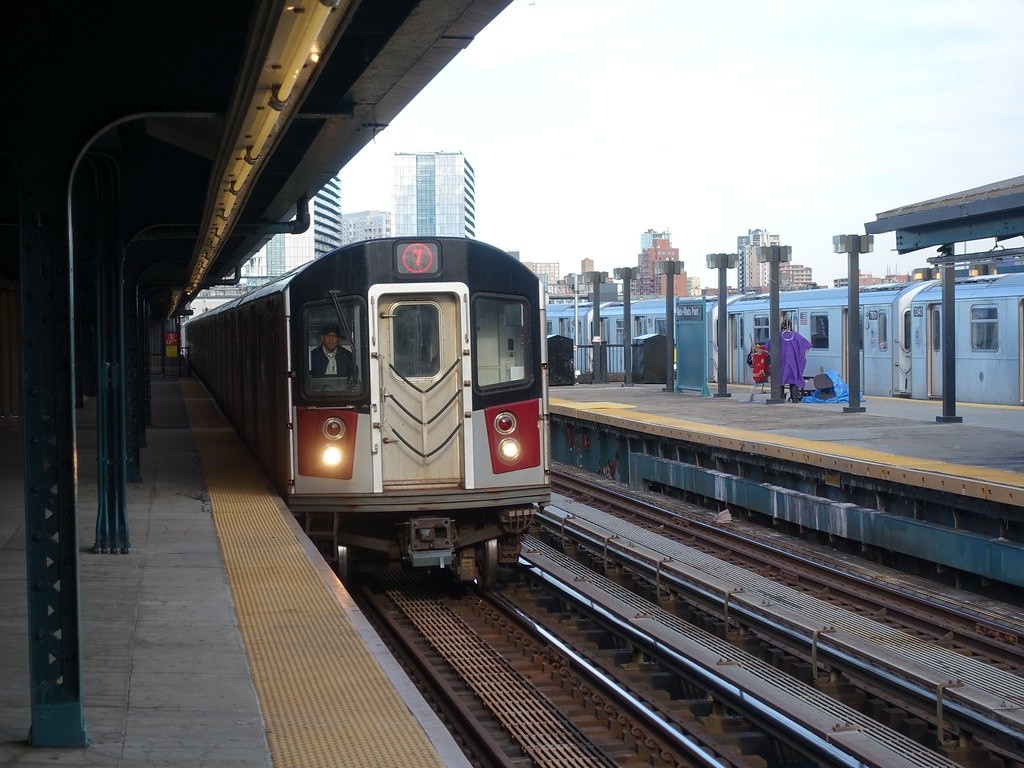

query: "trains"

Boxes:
[185, 235, 558, 601]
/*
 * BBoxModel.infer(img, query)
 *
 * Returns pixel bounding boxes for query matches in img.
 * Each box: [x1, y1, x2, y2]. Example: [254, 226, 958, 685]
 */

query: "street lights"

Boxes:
[584, 271, 609, 385]
[613, 267, 642, 387]
[756, 245, 793, 405]
[654, 260, 685, 392]
[706, 253, 736, 398]
[833, 234, 874, 413]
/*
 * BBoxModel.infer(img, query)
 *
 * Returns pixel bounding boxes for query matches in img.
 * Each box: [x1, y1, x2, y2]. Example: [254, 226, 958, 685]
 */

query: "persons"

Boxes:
[765, 321, 813, 403]
[311, 324, 353, 375]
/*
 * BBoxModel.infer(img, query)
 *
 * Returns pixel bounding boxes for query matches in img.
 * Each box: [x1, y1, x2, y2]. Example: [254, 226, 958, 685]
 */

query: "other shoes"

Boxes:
[792, 399, 799, 403]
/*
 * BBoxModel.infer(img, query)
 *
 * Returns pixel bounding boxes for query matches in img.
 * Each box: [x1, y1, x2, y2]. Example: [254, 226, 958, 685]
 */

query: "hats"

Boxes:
[322, 323, 339, 336]
